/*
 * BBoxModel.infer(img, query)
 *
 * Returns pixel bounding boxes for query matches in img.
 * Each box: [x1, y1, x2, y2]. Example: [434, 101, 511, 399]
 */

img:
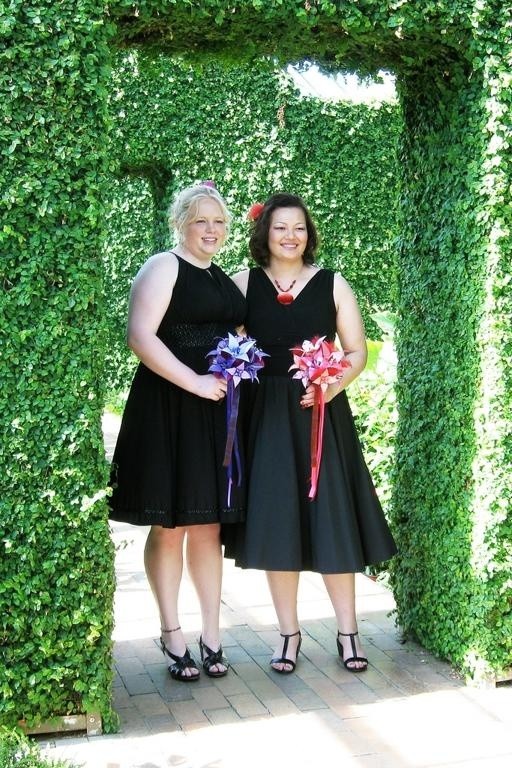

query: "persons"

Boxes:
[220, 191, 403, 676]
[104, 183, 251, 683]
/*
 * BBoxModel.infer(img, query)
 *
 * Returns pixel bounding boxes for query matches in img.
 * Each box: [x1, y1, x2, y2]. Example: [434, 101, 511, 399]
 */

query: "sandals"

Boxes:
[200, 635, 228, 677]
[336, 629, 368, 672]
[161, 637, 200, 682]
[271, 631, 302, 674]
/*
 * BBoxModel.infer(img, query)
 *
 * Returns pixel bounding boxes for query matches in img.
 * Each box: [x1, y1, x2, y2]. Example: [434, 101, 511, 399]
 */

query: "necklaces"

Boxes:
[269, 261, 303, 306]
